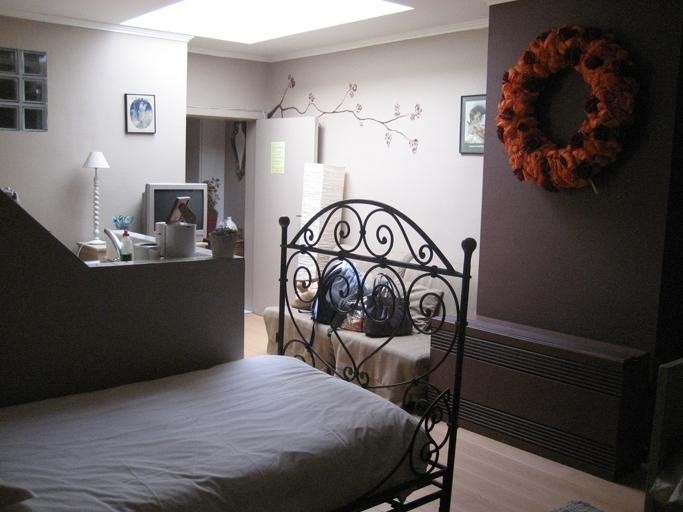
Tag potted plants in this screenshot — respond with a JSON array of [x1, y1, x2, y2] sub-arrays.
[[202, 178, 222, 236], [208, 227, 237, 259]]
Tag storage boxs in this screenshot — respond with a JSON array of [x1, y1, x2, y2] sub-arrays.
[[76, 241, 107, 262]]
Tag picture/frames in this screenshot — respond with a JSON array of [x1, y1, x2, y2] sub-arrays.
[[124, 93, 156, 134], [459, 94, 486, 155]]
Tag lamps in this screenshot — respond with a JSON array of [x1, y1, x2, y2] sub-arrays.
[[81, 150, 111, 245]]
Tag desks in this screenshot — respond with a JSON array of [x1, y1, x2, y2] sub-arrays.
[[204, 238, 244, 258]]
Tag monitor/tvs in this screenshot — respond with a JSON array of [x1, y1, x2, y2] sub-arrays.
[[146, 181, 208, 240]]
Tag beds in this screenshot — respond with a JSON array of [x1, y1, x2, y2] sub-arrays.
[[1, 198, 477, 508]]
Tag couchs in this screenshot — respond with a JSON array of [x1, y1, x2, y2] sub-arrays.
[[264, 271, 478, 410]]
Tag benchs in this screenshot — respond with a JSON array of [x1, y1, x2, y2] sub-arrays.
[[427, 313, 647, 486]]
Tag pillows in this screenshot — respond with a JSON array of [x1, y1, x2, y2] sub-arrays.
[[291, 282, 318, 311], [398, 281, 443, 334]]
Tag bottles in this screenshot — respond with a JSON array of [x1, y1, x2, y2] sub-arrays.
[[120, 229, 134, 262]]
